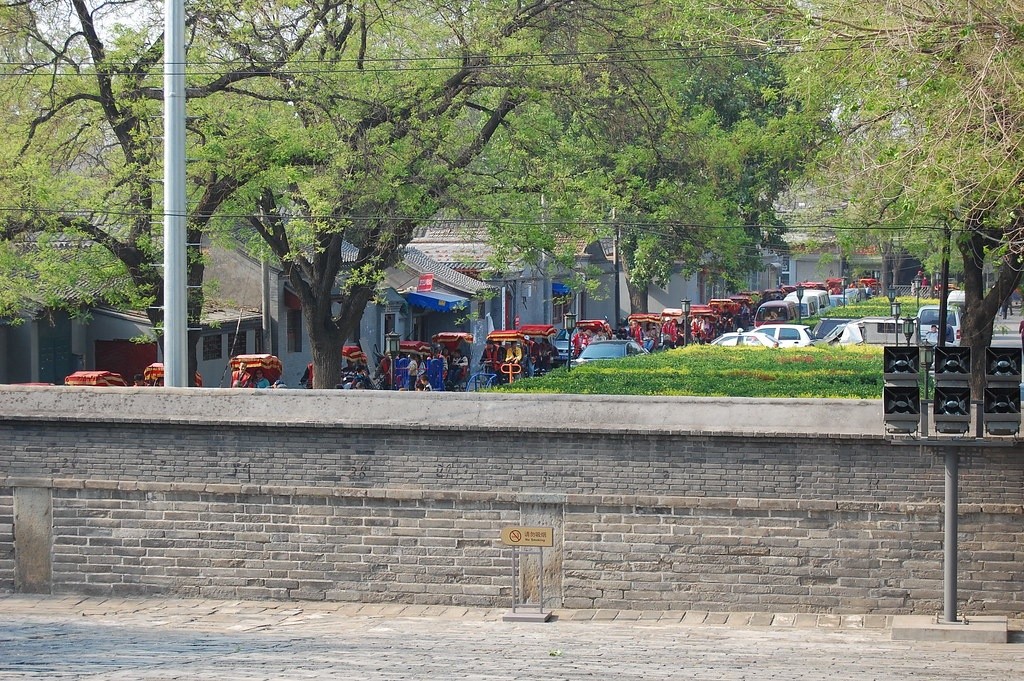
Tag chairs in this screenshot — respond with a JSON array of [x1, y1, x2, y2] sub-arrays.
[[925, 311, 936, 321]]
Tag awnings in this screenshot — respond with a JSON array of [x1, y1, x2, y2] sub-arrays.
[[408, 292, 469, 312], [552, 283, 571, 295]]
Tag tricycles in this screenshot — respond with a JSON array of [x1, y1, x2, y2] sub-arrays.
[[298, 278, 884, 392], [228, 354, 289, 389]]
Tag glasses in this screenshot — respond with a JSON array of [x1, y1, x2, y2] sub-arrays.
[[256, 372, 262, 375]]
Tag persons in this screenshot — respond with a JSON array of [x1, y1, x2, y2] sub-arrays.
[[496, 341, 507, 362], [519, 335, 539, 378], [535, 338, 555, 371], [925, 323, 954, 345], [911, 280, 916, 297], [421, 375, 432, 391], [446, 349, 468, 386], [480, 340, 506, 385], [341, 359, 355, 372], [406, 354, 425, 391], [999, 297, 1013, 319], [253, 369, 270, 388], [373, 352, 391, 390], [572, 280, 872, 359], [231, 362, 252, 388], [442, 347, 453, 365], [299, 363, 313, 389], [133, 374, 149, 386], [505, 341, 522, 364], [350, 368, 370, 389]]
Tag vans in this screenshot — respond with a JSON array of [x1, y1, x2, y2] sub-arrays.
[[947, 290, 966, 314], [913, 304, 963, 348], [751, 299, 798, 331], [783, 289, 831, 321]]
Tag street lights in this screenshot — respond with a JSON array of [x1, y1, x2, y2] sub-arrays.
[[794, 283, 805, 322], [873, 269, 881, 298], [679, 296, 692, 347], [841, 274, 849, 309], [934, 270, 939, 299], [900, 314, 915, 346], [890, 298, 902, 347], [563, 309, 579, 373], [913, 274, 923, 309]]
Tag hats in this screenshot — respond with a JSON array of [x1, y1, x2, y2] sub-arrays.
[[665, 317, 670, 321]]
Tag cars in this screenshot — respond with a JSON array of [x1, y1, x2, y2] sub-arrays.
[[748, 323, 817, 349], [707, 327, 784, 349], [570, 338, 648, 373], [551, 328, 580, 369], [841, 287, 865, 302], [828, 294, 849, 309]]
